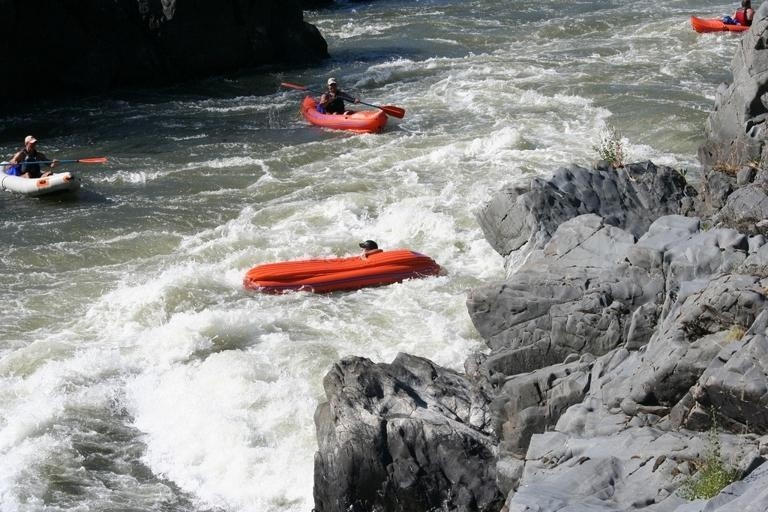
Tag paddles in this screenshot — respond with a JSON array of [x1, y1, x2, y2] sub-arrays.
[[0, 156, 107, 164], [281, 82, 405, 118]]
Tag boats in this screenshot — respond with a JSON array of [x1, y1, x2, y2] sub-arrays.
[[301, 95, 389, 134], [690, 12, 749, 33], [0, 160, 80, 194], [244, 249, 440, 293]]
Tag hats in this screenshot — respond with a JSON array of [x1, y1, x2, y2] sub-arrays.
[[359, 240, 378, 249], [327, 77, 337, 86], [25, 135, 37, 145]]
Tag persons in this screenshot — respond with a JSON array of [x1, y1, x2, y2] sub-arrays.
[[729, 0, 755, 27], [317, 76, 360, 115], [9, 134, 58, 178], [358, 240, 384, 261]]
